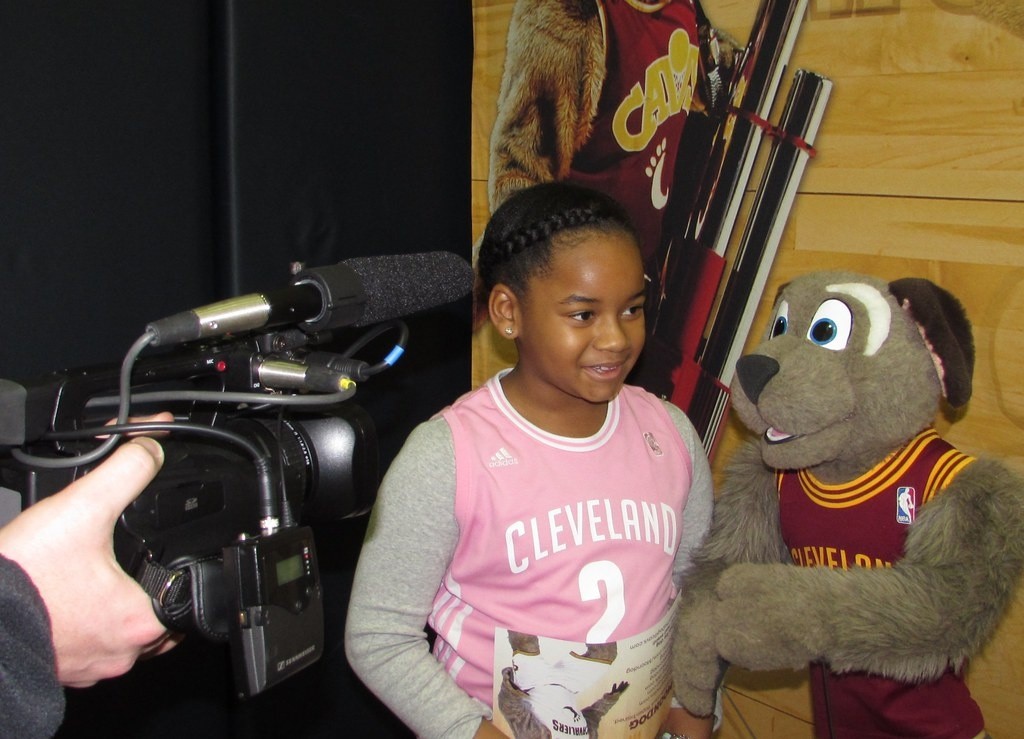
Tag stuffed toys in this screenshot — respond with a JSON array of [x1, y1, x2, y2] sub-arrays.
[[665, 269, 1024, 739]]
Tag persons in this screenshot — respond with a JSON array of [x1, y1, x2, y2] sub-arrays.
[[1, 411, 188, 739], [346, 179, 716, 739]]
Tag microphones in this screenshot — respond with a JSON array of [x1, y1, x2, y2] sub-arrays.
[[145, 250, 476, 347]]
